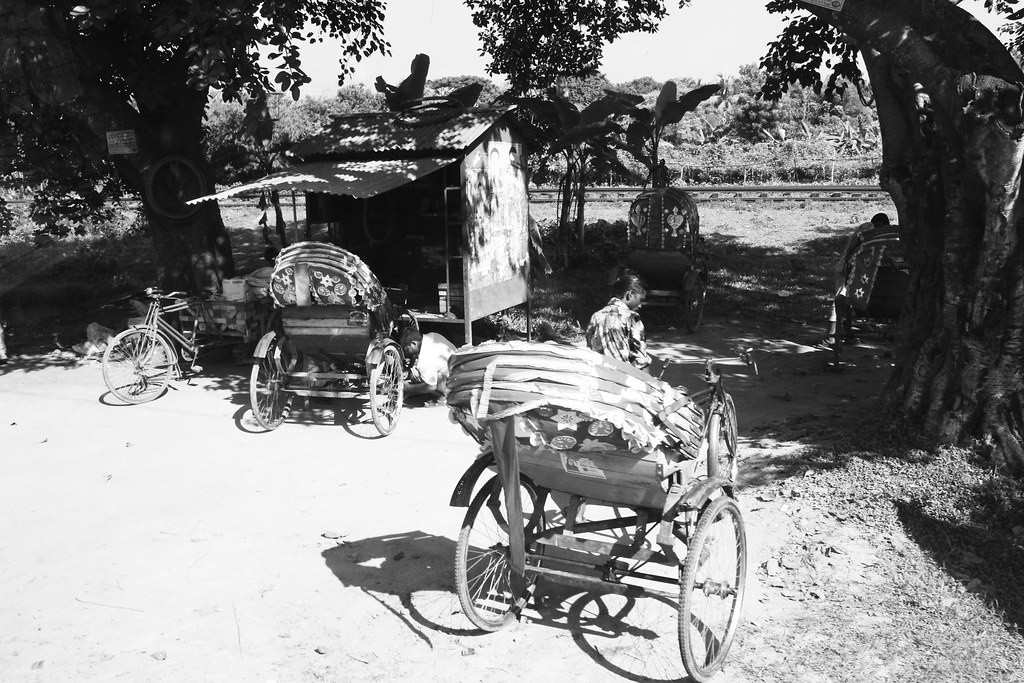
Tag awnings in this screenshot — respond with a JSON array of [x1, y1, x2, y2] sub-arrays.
[[184, 159, 458, 204]]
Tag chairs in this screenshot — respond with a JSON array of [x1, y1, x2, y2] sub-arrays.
[[624, 186, 710, 285], [843, 223, 910, 315], [268, 237, 404, 358], [446, 325, 712, 513]]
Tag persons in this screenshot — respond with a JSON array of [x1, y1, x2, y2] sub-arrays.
[[828, 213, 890, 346], [656, 158, 671, 188], [586, 274, 652, 374]]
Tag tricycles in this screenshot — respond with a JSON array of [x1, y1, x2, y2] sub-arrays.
[[615, 188, 712, 332], [828, 219, 909, 360], [242, 239, 422, 438], [416, 331, 761, 683], [101, 282, 285, 405]]
